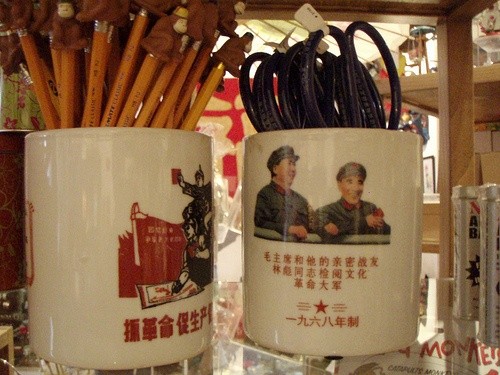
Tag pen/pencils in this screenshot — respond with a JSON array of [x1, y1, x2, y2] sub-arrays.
[[2, 1, 254, 130]]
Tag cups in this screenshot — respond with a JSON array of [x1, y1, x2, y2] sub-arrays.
[[23, 127, 215, 370], [242, 127, 423, 358]]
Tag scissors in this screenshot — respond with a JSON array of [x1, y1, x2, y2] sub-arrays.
[[238, 19, 402, 134]]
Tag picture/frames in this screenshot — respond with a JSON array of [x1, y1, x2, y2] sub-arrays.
[[423, 155, 436, 193]]
